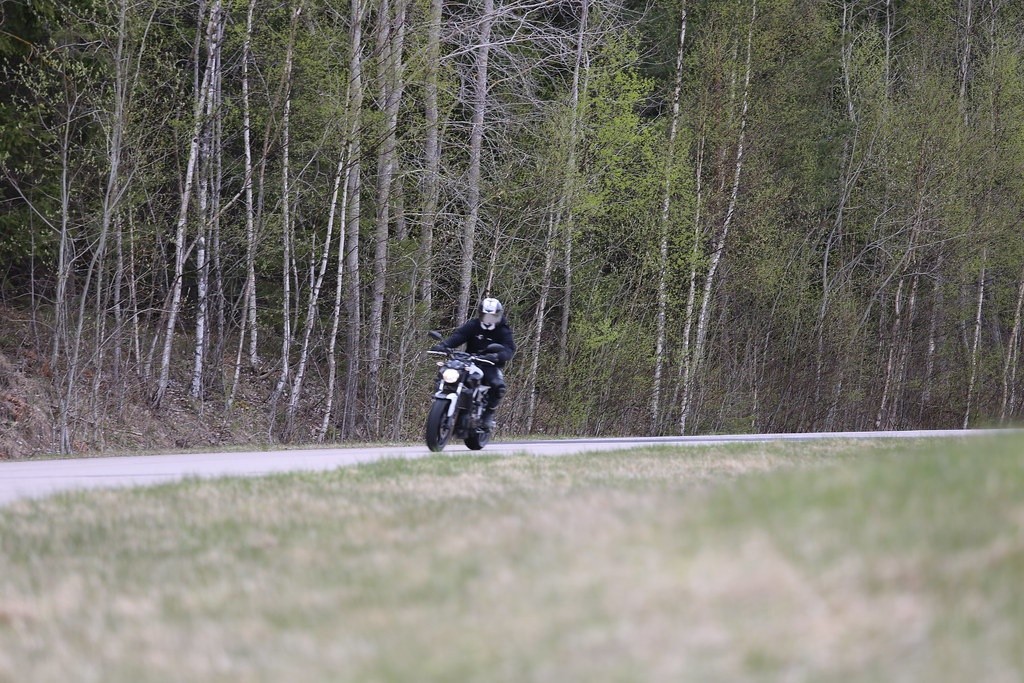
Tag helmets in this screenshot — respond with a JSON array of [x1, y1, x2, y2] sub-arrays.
[[478, 298, 504, 331]]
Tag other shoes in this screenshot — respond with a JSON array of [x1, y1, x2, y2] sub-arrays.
[[481, 411, 496, 429]]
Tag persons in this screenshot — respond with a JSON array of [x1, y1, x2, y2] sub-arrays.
[[430, 297, 516, 428]]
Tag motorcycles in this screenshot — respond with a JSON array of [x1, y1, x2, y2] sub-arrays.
[[426, 330, 506, 452]]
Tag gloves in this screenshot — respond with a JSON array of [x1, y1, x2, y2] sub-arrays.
[[430, 344, 449, 357], [479, 353, 499, 367]]
